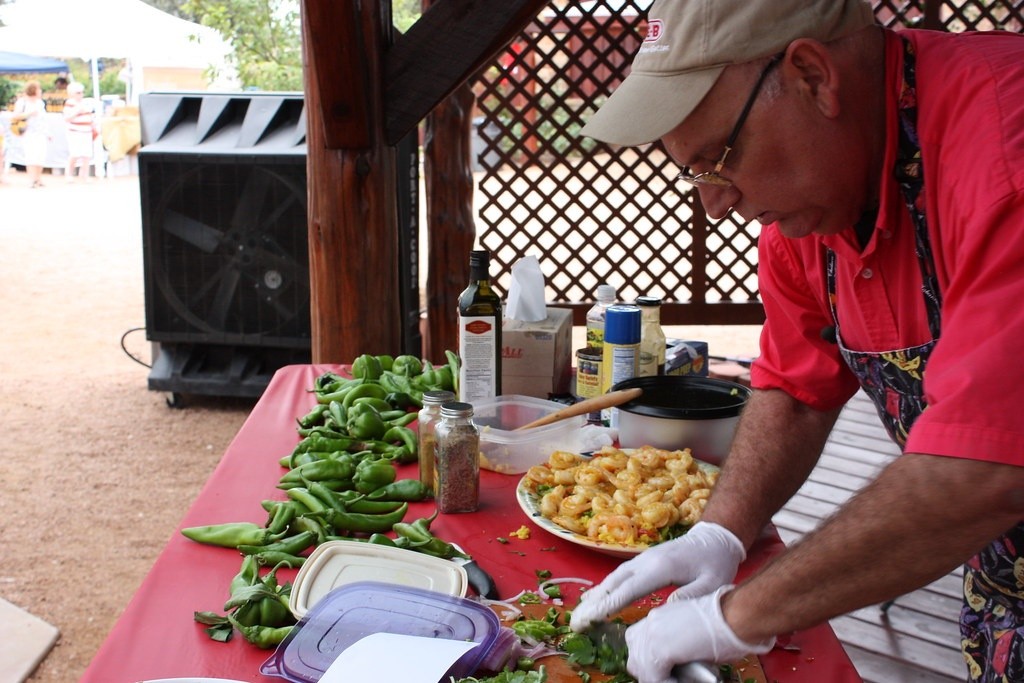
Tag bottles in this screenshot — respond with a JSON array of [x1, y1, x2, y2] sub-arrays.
[[636, 297, 666, 376], [434, 402, 479, 513], [457, 249, 502, 428], [585, 285, 616, 347], [417, 391, 456, 491]]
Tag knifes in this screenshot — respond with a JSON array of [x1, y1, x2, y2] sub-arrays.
[[587, 623, 721, 683]]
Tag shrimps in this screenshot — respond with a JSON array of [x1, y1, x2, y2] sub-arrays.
[[528, 445, 719, 545]]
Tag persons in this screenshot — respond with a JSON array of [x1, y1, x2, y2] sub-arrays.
[[61, 81, 93, 179], [570, 0, 1024, 683], [13, 81, 50, 189], [45, 76, 72, 113]]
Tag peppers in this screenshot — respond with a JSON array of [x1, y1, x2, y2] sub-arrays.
[[179, 351, 472, 648]]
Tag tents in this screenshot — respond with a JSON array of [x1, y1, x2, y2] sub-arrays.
[[0, 1, 222, 77]]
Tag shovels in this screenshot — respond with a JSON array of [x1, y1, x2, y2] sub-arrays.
[[478, 387, 641, 452]]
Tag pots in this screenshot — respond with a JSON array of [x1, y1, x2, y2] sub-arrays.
[[606, 375, 753, 465]]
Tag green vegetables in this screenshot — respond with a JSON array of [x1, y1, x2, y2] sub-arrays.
[[449, 518, 760, 683]]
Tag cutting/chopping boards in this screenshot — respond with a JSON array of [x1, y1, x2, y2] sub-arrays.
[[478, 600, 767, 683]]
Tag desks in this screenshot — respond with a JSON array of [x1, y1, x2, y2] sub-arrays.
[[74, 365, 861, 683]]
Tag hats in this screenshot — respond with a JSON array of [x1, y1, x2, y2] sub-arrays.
[[579, 0, 875, 147]]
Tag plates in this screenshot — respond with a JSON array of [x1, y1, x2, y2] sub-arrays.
[[515, 448, 721, 558]]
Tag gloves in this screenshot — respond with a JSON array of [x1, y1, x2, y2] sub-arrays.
[[625, 584, 777, 683], [570, 521, 747, 634]]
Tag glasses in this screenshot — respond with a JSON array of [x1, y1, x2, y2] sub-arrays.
[[678, 43, 792, 193]]
